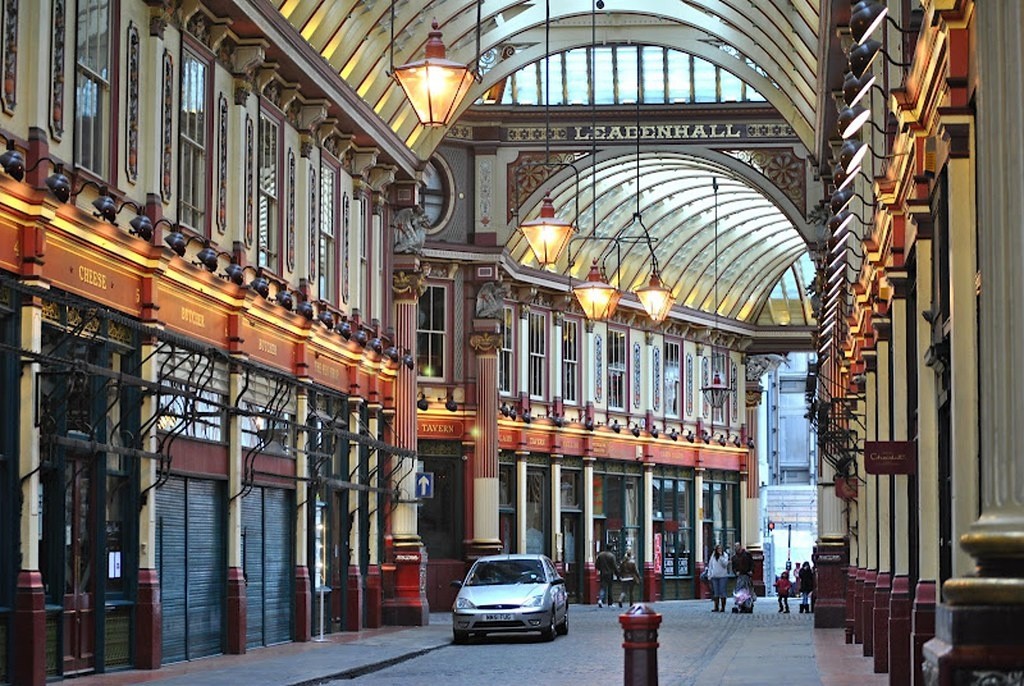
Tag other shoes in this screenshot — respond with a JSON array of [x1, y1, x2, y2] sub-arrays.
[[618, 599, 623, 608], [608, 605, 616, 609], [779, 609, 783, 612], [784, 611, 789, 613], [598, 600, 603, 608]]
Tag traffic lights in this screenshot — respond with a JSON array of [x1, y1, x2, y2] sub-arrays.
[[768, 522, 775, 531]]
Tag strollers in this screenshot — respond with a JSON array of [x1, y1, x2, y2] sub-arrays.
[[731, 572, 757, 614]]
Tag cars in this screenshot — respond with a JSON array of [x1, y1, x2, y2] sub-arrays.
[[450, 553, 568, 644]]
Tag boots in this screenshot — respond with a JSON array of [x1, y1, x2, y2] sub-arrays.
[[711, 597, 719, 612], [800, 604, 804, 614], [720, 597, 726, 612], [804, 604, 810, 613]]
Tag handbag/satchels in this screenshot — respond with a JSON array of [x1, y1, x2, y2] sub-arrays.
[[699, 567, 709, 583]]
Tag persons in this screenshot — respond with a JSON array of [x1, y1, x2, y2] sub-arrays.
[[773, 570, 791, 613], [708, 545, 728, 612], [798, 561, 813, 613], [595, 543, 640, 608], [731, 542, 756, 602]]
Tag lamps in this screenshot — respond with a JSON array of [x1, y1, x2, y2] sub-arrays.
[[804, 0, 920, 494], [415, 391, 756, 450], [1, 132, 414, 372], [695, 177, 732, 408], [512, 0, 581, 265], [567, 0, 624, 321], [633, 43, 676, 322], [384, 0, 484, 130]]
